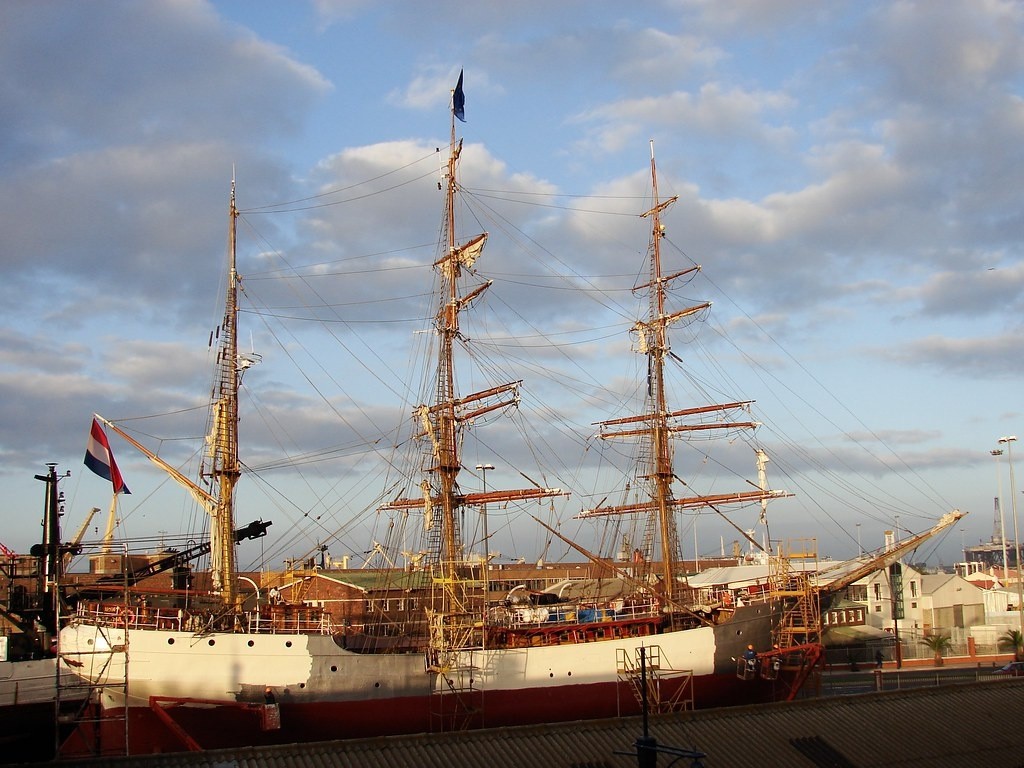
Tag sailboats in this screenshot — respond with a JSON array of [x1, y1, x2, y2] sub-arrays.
[[44, 64, 972, 755]]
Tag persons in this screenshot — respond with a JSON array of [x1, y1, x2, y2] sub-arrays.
[[769, 644, 784, 678], [875, 649, 885, 669], [744, 644, 757, 671]]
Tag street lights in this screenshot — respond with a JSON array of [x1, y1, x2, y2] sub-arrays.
[[894, 515, 901, 543], [989, 448, 1009, 590], [856, 522, 862, 558], [997, 434, 1024, 643], [475, 463, 497, 623], [959, 528, 968, 561]]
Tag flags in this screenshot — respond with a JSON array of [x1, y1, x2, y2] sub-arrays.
[[448, 67, 466, 123], [83, 414, 132, 494]]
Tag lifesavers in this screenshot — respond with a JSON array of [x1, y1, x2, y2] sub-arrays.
[[118, 610, 135, 624]]
